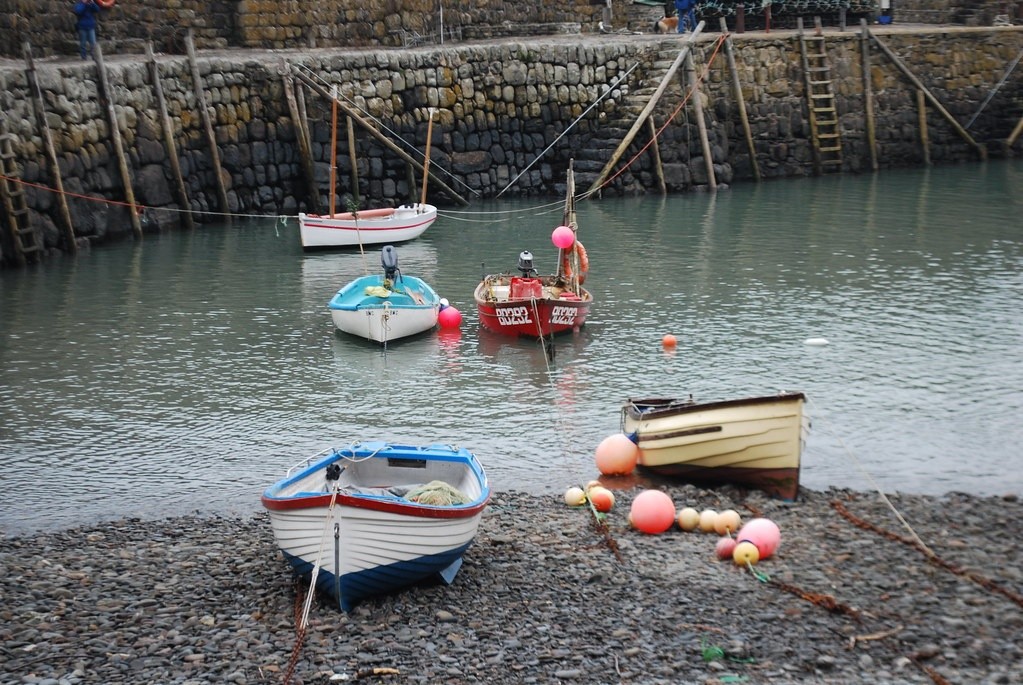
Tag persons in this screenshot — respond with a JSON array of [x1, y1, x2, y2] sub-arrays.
[[73, 0, 99, 62], [675, 0, 698, 34]]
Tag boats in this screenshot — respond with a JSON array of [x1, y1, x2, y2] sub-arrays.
[[621, 393, 809, 502], [474, 250, 594, 338], [261, 441, 491, 612], [329, 246, 441, 342], [298, 203, 438, 254]]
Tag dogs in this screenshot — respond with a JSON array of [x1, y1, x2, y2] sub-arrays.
[[657, 16, 679, 35]]
